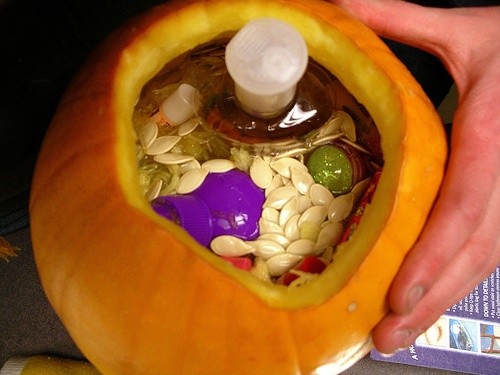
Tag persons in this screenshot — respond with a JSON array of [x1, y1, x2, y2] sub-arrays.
[[319, 1, 499, 354]]
